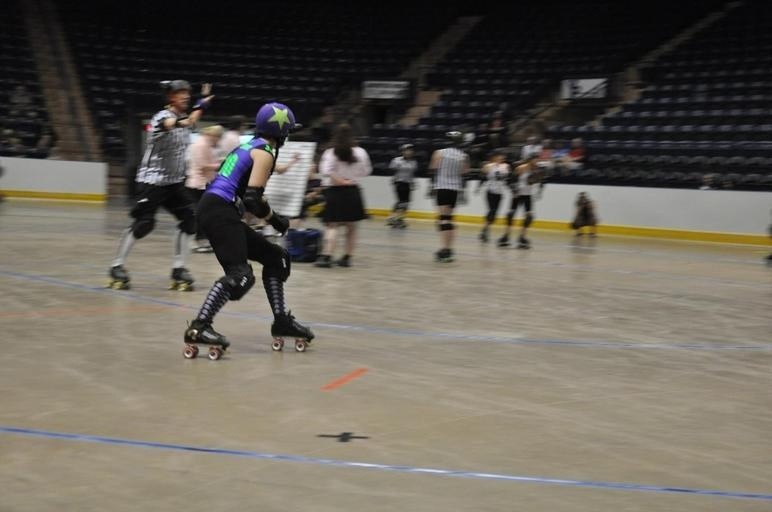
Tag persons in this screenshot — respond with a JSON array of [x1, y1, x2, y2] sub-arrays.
[[316, 121, 372, 268], [572, 192, 596, 237], [185, 115, 300, 226], [184, 102, 314, 360], [475, 147, 539, 249], [3, 85, 50, 150], [427, 132, 470, 265], [387, 143, 418, 230], [522, 136, 584, 183], [107, 81, 214, 288]]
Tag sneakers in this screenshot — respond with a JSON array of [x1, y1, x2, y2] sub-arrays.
[[589, 232, 598, 239], [336, 253, 354, 267], [576, 232, 585, 238], [315, 253, 333, 269]]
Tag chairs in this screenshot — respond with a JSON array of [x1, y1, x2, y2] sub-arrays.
[[0, 1, 772, 193]]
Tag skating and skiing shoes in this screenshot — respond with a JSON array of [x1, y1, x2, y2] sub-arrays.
[[496, 232, 512, 249], [434, 248, 454, 265], [386, 214, 409, 231], [270, 314, 316, 353], [107, 264, 132, 292], [168, 267, 197, 294], [182, 321, 230, 360], [515, 235, 533, 250]]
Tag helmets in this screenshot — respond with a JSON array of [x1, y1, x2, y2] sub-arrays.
[[164, 78, 193, 97], [400, 142, 415, 151], [446, 130, 463, 139], [254, 101, 302, 147]]
